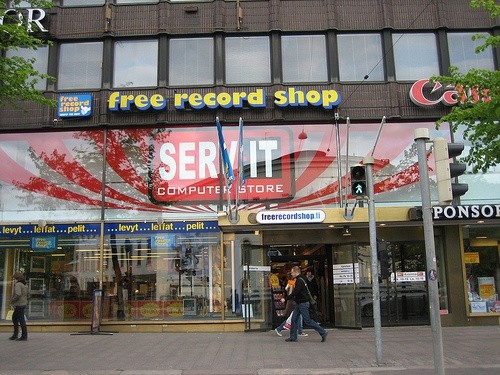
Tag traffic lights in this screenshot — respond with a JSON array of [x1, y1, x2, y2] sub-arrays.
[[434, 137, 471, 203], [350, 166, 368, 198]]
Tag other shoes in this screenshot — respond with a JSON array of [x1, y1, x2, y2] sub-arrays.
[[275, 329, 283, 337], [9, 335, 18, 340], [16, 336, 27, 341], [298, 333, 309, 337], [285, 338, 297, 342], [321, 331, 328, 342]]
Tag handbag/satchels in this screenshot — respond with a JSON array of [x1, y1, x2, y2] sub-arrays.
[[310, 297, 318, 305], [6, 309, 15, 321], [283, 311, 294, 331]]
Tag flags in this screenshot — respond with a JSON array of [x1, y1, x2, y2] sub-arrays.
[[216, 121, 245, 186]]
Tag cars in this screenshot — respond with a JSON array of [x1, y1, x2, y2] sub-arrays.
[[361, 289, 428, 317]]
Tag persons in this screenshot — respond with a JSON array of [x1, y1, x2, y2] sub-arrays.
[[66, 277, 84, 301], [9, 271, 29, 341], [306, 268, 322, 325], [274, 272, 309, 337], [280, 266, 328, 342]]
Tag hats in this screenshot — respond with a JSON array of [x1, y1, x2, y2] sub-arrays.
[[13, 272, 25, 282]]
